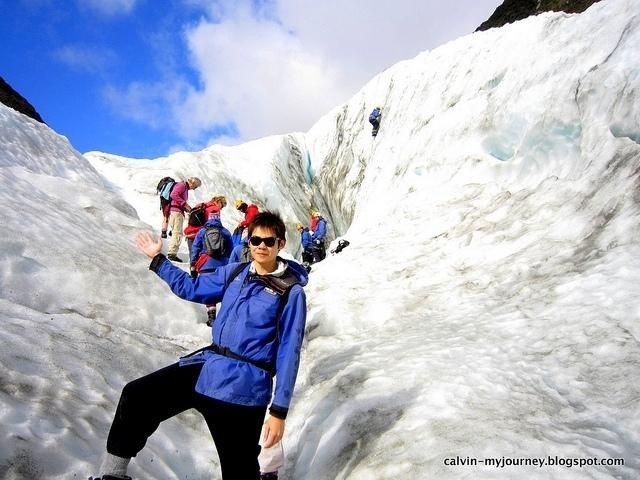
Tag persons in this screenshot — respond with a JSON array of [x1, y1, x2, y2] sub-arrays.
[[183, 195, 227, 262], [168, 176, 202, 262], [296, 224, 314, 265], [369, 106, 381, 136], [190, 209, 234, 327], [94, 206, 309, 479], [235, 200, 259, 229], [161, 203, 172, 238], [312, 212, 328, 263]]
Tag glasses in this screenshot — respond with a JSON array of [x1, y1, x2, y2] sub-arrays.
[[248, 237, 281, 247]]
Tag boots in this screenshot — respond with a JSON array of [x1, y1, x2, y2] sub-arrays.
[[206, 310, 216, 326]]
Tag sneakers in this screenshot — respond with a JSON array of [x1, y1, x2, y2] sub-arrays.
[[162, 231, 172, 238]]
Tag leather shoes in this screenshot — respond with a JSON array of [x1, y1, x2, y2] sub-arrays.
[[168, 254, 183, 262]]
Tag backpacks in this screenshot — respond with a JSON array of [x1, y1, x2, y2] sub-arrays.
[[188, 203, 206, 226], [157, 177, 186, 210], [203, 227, 228, 259]]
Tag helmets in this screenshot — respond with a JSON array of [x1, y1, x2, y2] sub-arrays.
[[236, 200, 245, 209], [298, 224, 303, 230], [314, 212, 321, 217]]
